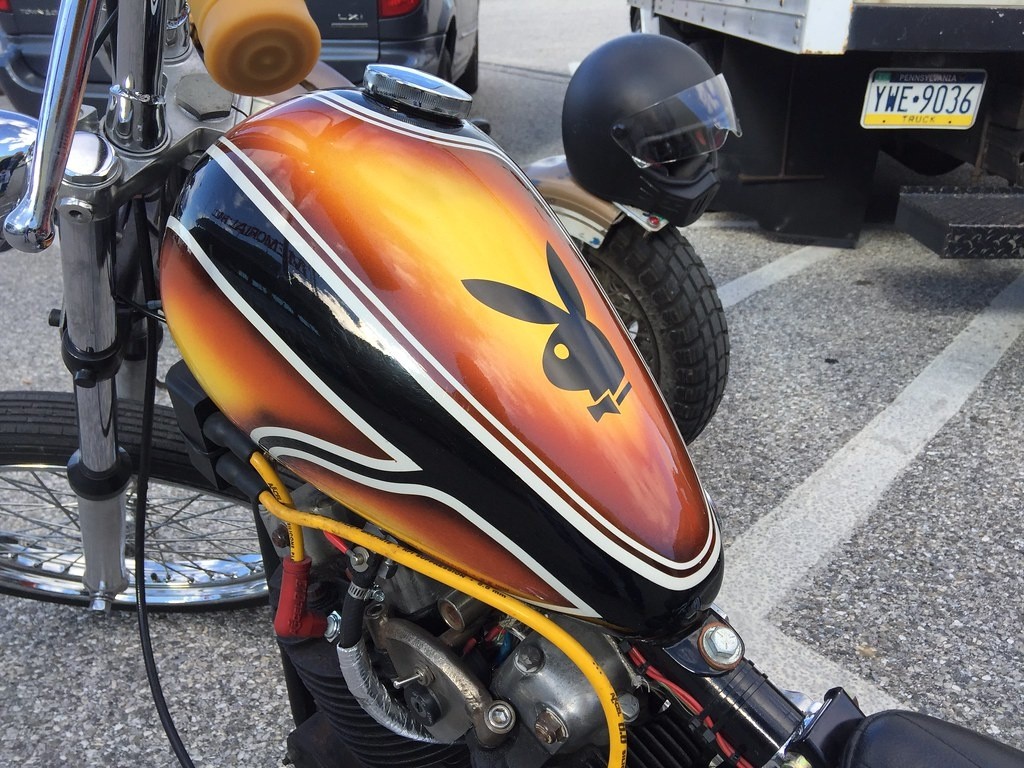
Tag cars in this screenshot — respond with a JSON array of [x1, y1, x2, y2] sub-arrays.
[[0, 3, 481, 124]]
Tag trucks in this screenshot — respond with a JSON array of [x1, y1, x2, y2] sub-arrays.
[[629, 1, 1024, 261]]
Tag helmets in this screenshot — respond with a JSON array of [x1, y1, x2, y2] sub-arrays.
[[561, 33, 744, 228]]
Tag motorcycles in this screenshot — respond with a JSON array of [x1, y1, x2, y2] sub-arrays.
[[0, 1, 1024, 768]]
